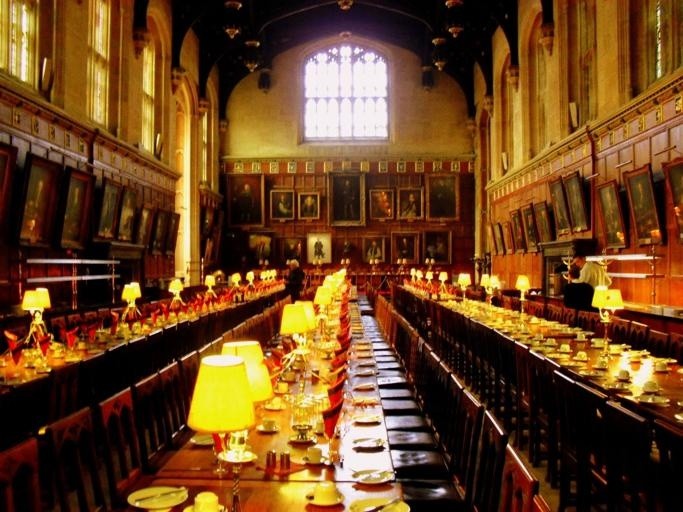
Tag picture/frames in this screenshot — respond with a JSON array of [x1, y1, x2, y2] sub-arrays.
[[0, 142, 181, 258], [218, 160, 472, 265], [485, 157, 683, 257]]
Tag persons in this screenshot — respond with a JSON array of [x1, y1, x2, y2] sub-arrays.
[[65, 185, 82, 218], [287, 239, 295, 257], [634, 180, 650, 217], [301, 196, 316, 217], [603, 186, 619, 234], [97, 191, 114, 237], [119, 198, 133, 240], [239, 183, 251, 201], [313, 237, 322, 259], [570, 182, 584, 226], [340, 179, 354, 219], [538, 209, 548, 240], [514, 216, 523, 249], [400, 192, 416, 216], [567, 252, 611, 290], [367, 240, 381, 258], [430, 178, 455, 218], [31, 179, 43, 201], [273, 192, 291, 217], [279, 259, 305, 303], [559, 265, 598, 313], [295, 240, 303, 258], [397, 236, 413, 259]]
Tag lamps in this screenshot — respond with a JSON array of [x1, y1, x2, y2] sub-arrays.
[[419, 0, 462, 89], [219, 0, 276, 96]]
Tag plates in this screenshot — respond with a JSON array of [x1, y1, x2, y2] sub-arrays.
[[436, 300, 683, 429], [0, 280, 409, 511]]
